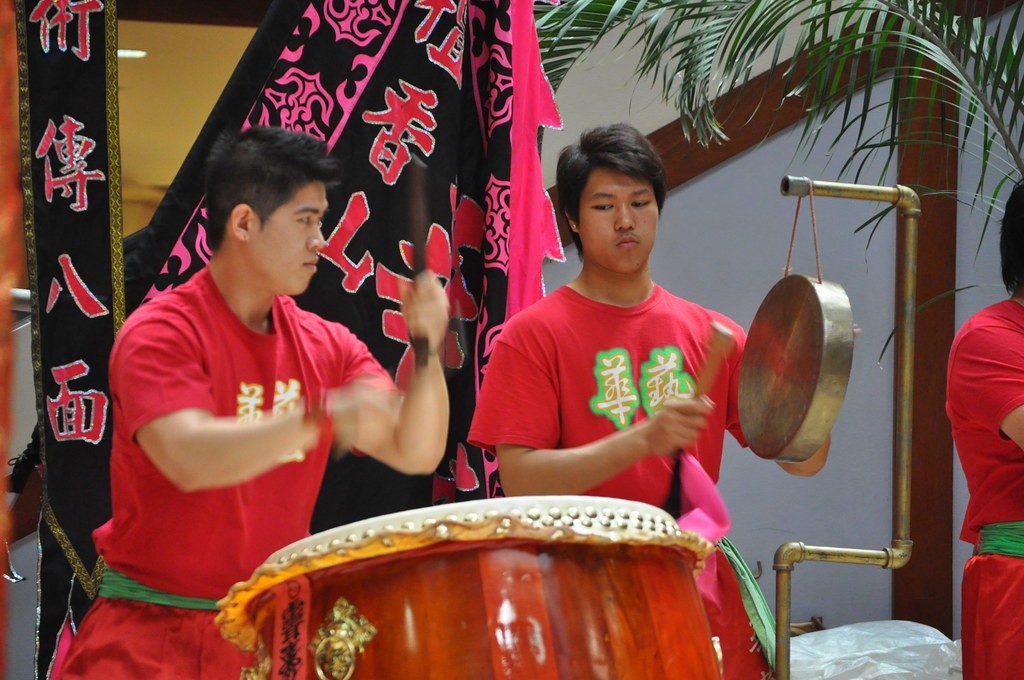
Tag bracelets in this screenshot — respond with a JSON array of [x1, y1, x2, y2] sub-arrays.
[[430, 349, 438, 357]]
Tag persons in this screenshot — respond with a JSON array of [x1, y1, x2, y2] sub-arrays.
[[946, 177, 1024, 680], [60, 123, 451, 680], [466, 121, 862, 680]]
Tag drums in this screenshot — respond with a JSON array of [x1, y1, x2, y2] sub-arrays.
[[217, 496, 728, 680]]
